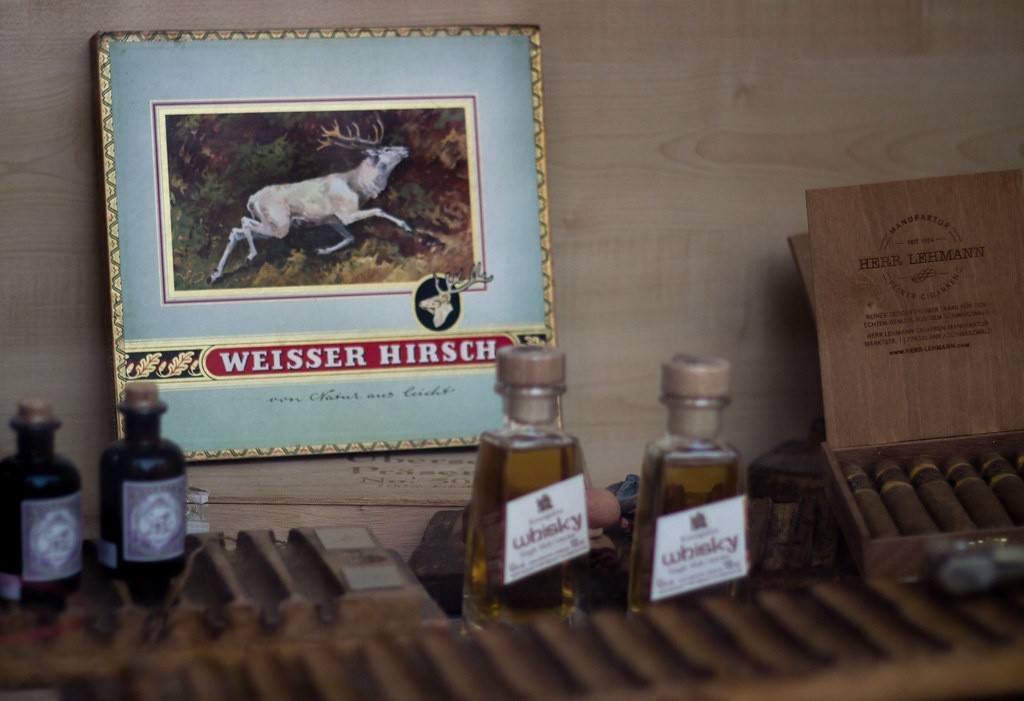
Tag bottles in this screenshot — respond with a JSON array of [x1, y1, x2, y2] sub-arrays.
[[96, 384, 187, 582], [464, 346, 587, 629], [628, 354, 749, 614], [1, 401, 82, 602]]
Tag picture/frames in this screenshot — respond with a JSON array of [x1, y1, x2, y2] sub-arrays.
[[89, 23, 562, 462]]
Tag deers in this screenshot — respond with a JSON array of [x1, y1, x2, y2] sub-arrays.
[[207, 116, 413, 284]]
